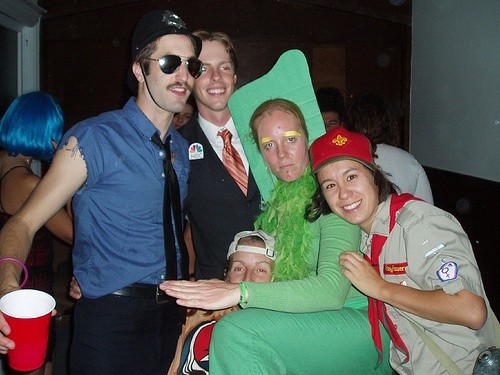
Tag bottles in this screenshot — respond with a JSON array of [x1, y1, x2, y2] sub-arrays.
[[473, 349, 500, 375]]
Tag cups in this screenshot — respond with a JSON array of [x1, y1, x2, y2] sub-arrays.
[[0, 289, 56, 371]]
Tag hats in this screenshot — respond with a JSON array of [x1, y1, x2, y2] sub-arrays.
[[226, 229, 277, 262], [126, 8, 202, 85], [307, 126, 377, 176]]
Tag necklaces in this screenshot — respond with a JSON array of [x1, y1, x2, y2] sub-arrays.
[[26, 159, 30, 167]]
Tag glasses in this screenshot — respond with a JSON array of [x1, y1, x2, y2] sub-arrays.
[[140, 53, 205, 80]]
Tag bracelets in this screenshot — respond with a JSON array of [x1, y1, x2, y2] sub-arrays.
[[238, 282, 246, 305], [0, 257, 30, 287]]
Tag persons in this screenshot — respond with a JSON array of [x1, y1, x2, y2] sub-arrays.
[[160, 49, 394, 375], [179, 30, 268, 282], [0, 9, 203, 375], [304, 126, 500, 375], [70, 88, 435, 375], [0, 91, 74, 375]]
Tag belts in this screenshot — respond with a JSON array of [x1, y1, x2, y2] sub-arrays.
[[109, 282, 178, 305]]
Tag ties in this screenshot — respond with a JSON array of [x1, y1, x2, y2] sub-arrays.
[[216, 127, 249, 198], [151, 131, 191, 282], [363, 191, 427, 366]]
